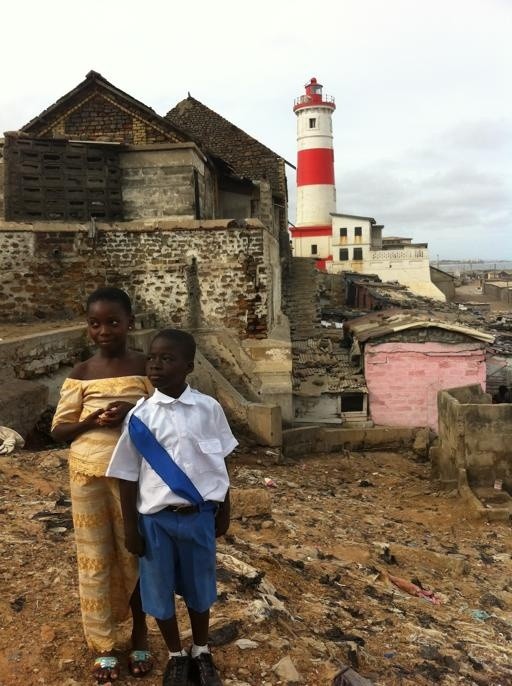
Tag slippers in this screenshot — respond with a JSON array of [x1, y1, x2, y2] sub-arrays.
[[94, 651, 152, 681]]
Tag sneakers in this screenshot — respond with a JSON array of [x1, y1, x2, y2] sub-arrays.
[[164, 653, 223, 686]]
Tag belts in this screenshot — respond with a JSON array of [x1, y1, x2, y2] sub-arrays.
[[169, 505, 199, 514]]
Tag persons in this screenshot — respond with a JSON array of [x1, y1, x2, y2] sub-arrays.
[[50, 285, 157, 683], [104, 327, 242, 686]]
